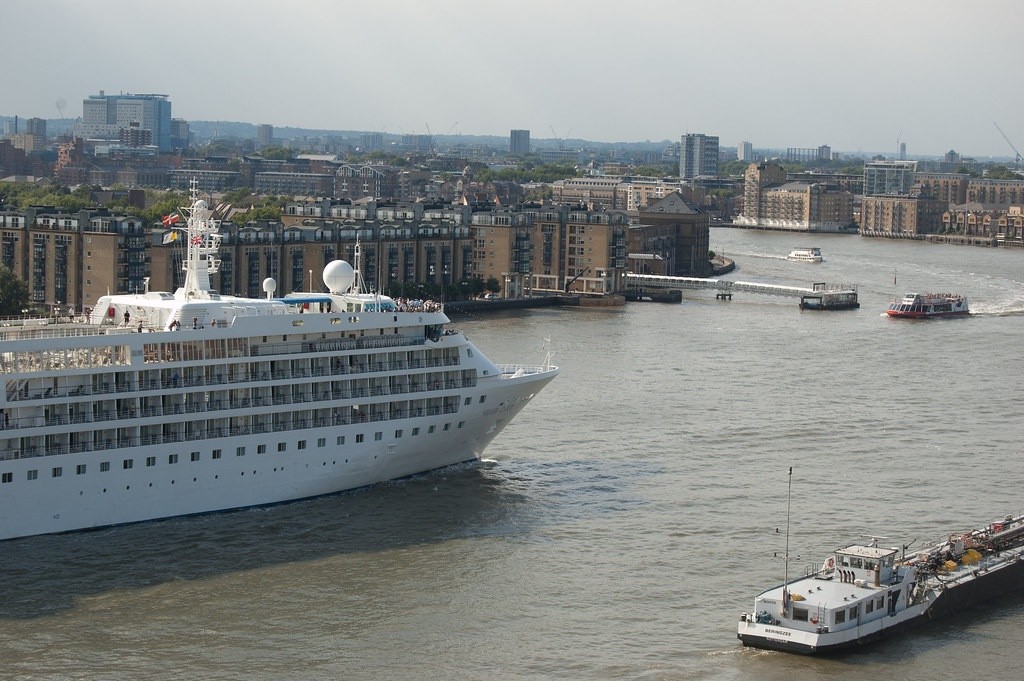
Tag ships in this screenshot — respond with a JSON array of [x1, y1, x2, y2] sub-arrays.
[[2, 185, 561, 546]]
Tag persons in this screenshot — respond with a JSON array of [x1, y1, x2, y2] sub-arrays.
[[175, 321, 182, 331], [387, 294, 458, 342], [210, 318, 216, 328], [172, 372, 181, 389], [915, 292, 966, 303], [192, 316, 198, 329], [168, 320, 175, 332], [356, 410, 366, 424], [336, 414, 346, 424], [83, 308, 93, 325], [137, 319, 143, 332], [67, 306, 77, 323], [4, 412, 10, 430], [336, 358, 344, 373], [434, 378, 440, 391], [123, 310, 131, 326]]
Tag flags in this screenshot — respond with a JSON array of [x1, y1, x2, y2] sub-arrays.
[[162, 231, 177, 245], [190, 235, 205, 247], [162, 210, 180, 228]]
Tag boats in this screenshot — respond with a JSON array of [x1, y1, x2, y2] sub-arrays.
[[738, 465, 1024, 659], [798, 287, 861, 311], [788, 246, 824, 263], [886, 291, 972, 319]]
